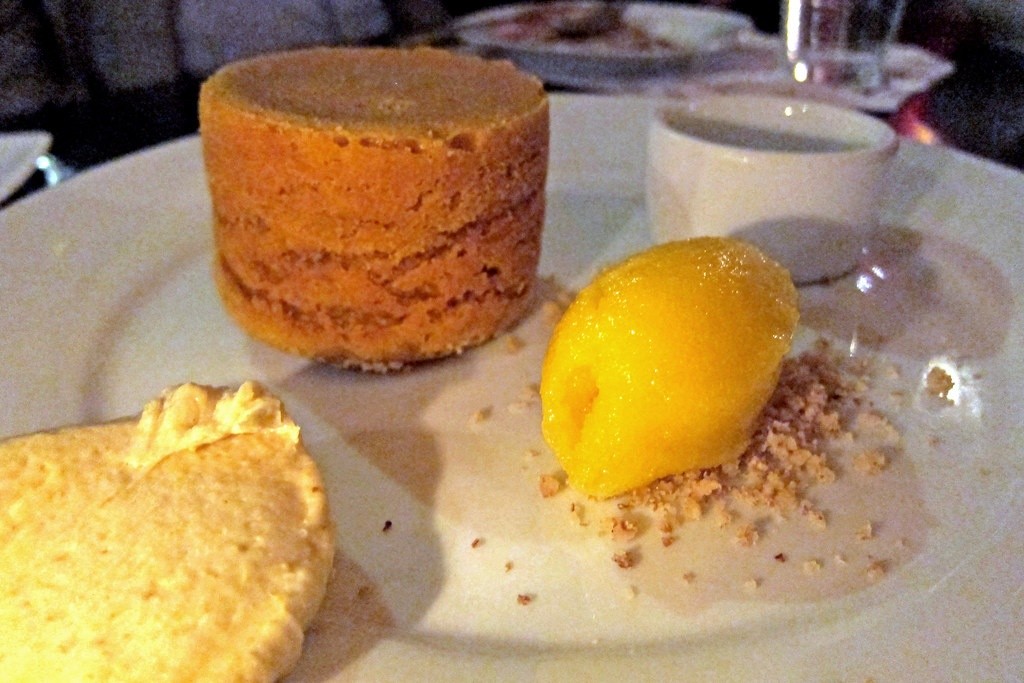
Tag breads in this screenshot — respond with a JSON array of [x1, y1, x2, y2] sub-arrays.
[[200, 46, 548, 367]]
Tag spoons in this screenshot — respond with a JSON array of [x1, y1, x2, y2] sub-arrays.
[[398, 3, 626, 46]]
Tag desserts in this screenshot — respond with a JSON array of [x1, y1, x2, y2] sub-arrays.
[[540, 234, 802, 501]]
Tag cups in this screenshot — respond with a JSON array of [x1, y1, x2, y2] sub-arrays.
[[780, 0, 905, 96]]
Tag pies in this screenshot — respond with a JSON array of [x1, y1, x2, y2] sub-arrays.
[[0, 374, 338, 683]]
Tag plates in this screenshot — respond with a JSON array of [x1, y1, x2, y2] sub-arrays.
[[0, 92, 1024, 682], [0, 131, 53, 203], [451, 1, 751, 72]]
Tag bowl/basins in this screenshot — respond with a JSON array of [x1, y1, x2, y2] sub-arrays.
[[645, 93, 899, 283]]
